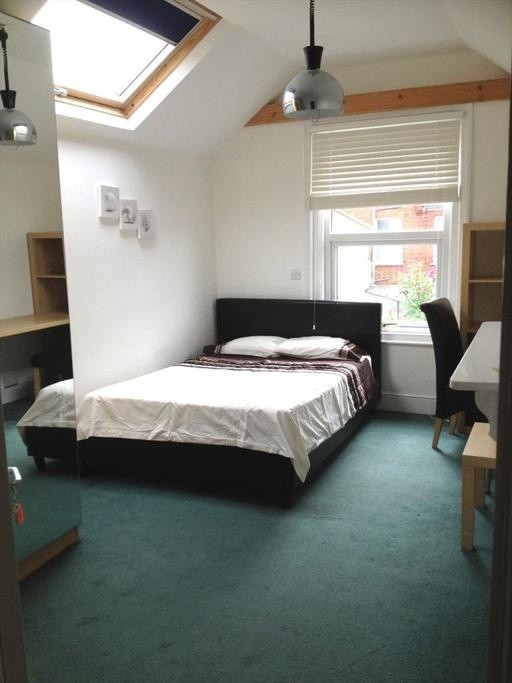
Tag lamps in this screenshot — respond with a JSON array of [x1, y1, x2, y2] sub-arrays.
[[281, 0, 346, 123], [0, 22, 39, 148]]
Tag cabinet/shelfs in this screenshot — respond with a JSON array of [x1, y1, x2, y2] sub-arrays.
[[460, 220, 506, 361], [25, 231, 69, 384]]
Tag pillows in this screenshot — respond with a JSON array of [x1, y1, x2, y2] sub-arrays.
[[203, 331, 368, 363]]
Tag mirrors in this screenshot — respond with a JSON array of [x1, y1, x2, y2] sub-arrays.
[[0, 9, 84, 565]]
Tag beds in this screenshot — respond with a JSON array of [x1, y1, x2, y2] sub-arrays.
[[73, 296, 382, 510]]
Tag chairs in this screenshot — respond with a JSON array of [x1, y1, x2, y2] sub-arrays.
[[419, 296, 476, 450]]
